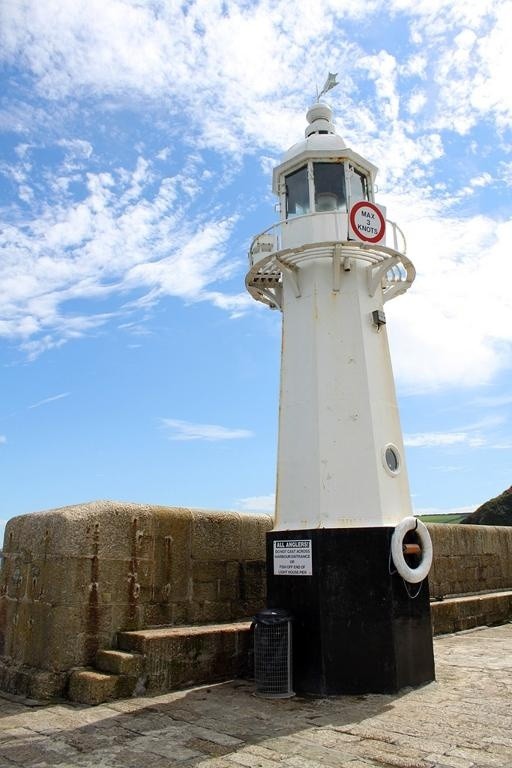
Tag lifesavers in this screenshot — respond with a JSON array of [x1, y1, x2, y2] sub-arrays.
[[391, 517, 432, 582]]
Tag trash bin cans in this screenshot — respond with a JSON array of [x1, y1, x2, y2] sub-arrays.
[[250, 608, 296, 699]]
